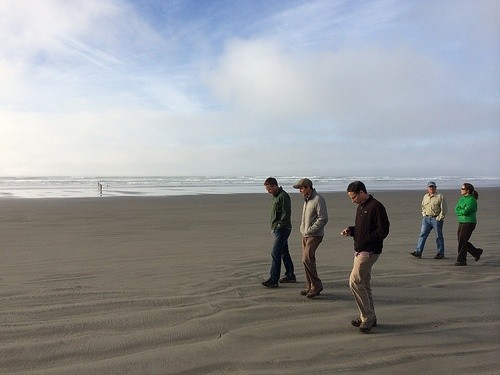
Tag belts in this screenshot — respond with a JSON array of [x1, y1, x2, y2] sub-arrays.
[[426, 215, 437, 217]]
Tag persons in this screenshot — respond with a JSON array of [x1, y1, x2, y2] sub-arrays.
[[342, 181, 390, 332], [454, 183, 483, 267], [262, 177, 296, 287], [293, 178, 323, 297], [412, 182, 447, 259]]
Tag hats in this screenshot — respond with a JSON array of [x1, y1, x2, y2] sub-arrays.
[[428, 182, 436, 187], [293, 178, 312, 189]]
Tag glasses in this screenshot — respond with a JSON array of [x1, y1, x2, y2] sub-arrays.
[[461, 188, 464, 190]]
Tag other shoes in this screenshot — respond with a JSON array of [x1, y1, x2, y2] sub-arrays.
[[360, 316, 375, 332], [411, 251, 421, 257], [279, 277, 296, 283], [300, 289, 320, 295], [434, 253, 444, 258], [262, 281, 278, 286], [475, 249, 483, 261], [306, 288, 323, 297], [455, 262, 466, 266], [351, 315, 376, 327]]
